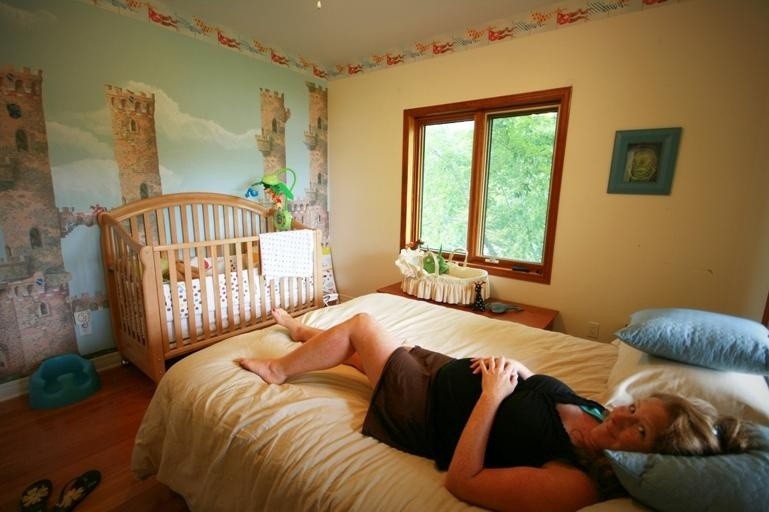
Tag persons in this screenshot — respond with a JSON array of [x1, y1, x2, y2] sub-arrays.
[[236, 307, 749, 511]]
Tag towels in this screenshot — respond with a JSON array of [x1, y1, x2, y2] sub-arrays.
[[257, 227, 315, 287]]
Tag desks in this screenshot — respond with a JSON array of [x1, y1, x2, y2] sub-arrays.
[[377, 280, 560, 331]]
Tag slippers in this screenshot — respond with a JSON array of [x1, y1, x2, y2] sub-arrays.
[[20, 479, 53, 512], [51, 469, 102, 512]]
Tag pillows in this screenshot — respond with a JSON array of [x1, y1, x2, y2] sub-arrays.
[[599, 303, 769, 512], [108, 253, 170, 281]]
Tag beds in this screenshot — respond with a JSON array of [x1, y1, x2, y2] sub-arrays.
[[128, 288, 769, 512], [95, 191, 324, 390]]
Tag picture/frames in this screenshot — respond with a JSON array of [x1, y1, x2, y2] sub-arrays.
[[606, 123, 684, 198]]
